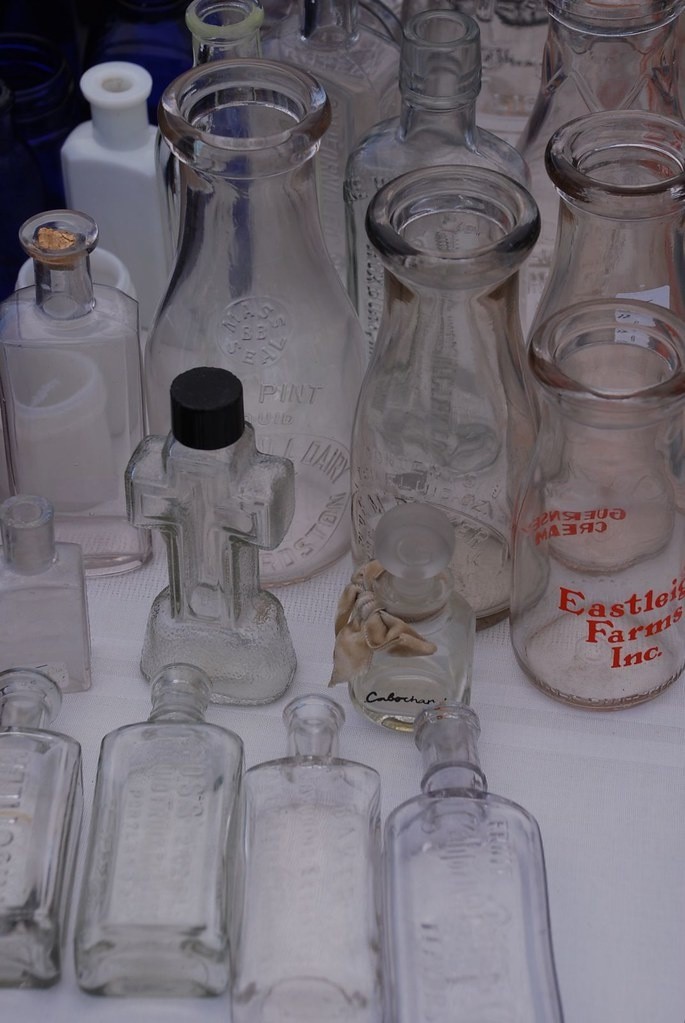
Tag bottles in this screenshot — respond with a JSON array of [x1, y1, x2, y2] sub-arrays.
[[0, 668, 83, 989], [72, 662, 243, 998], [232, 695, 384, 1023], [0, 0, 685, 735], [377, 703, 564, 1022]]
